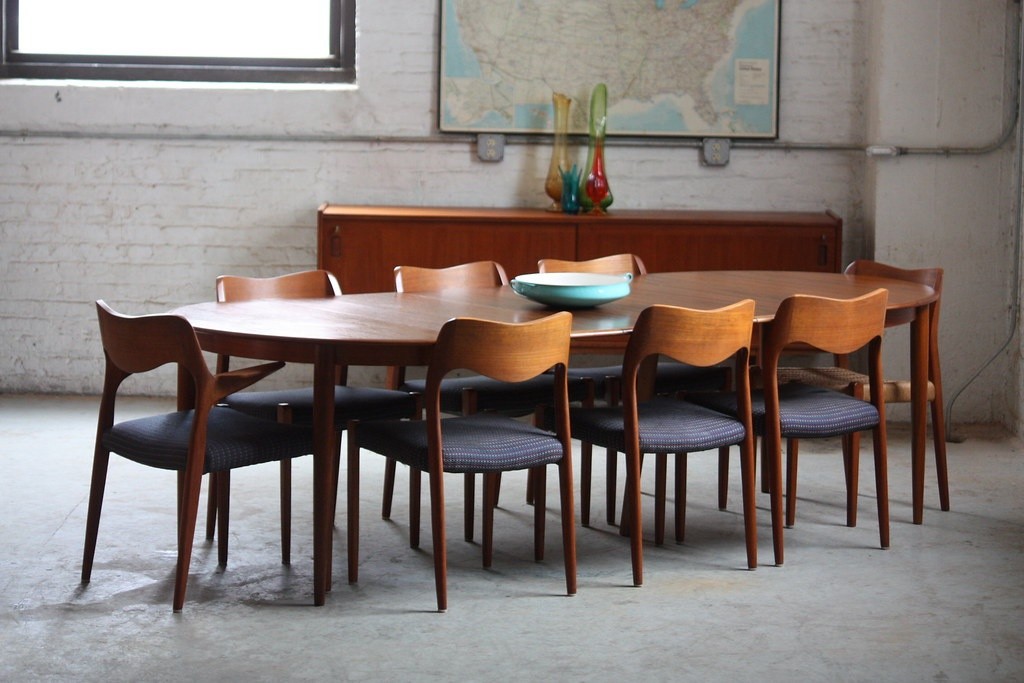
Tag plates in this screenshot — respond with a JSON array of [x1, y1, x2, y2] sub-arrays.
[[510, 271, 633, 306]]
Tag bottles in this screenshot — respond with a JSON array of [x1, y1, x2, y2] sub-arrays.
[[545, 82, 614, 217]]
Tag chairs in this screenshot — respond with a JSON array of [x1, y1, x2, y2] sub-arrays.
[[837, 257, 951, 523], [80, 250, 886, 615]]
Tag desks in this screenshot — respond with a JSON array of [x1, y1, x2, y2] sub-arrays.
[[165, 269, 938, 608], [315, 202, 844, 275]]
